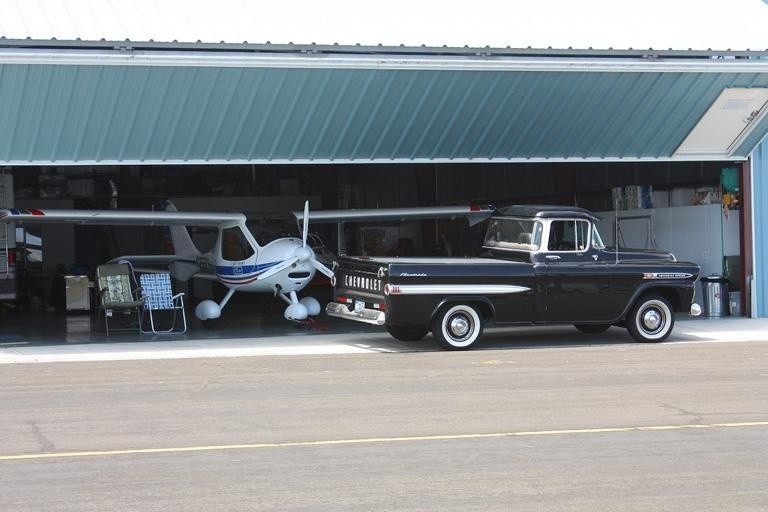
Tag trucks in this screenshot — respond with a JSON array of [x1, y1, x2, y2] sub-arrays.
[[0, 166, 17, 300]]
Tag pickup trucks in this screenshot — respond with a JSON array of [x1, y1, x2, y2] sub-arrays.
[[325, 204, 702, 350]]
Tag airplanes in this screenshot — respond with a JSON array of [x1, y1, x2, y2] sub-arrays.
[[0, 176, 497, 330]]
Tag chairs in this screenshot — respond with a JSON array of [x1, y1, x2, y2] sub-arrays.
[[92, 263, 189, 337]]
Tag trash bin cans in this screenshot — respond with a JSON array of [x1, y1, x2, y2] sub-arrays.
[[700, 273, 728, 317]]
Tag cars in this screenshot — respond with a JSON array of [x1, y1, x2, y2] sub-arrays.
[[17, 243, 41, 300]]
